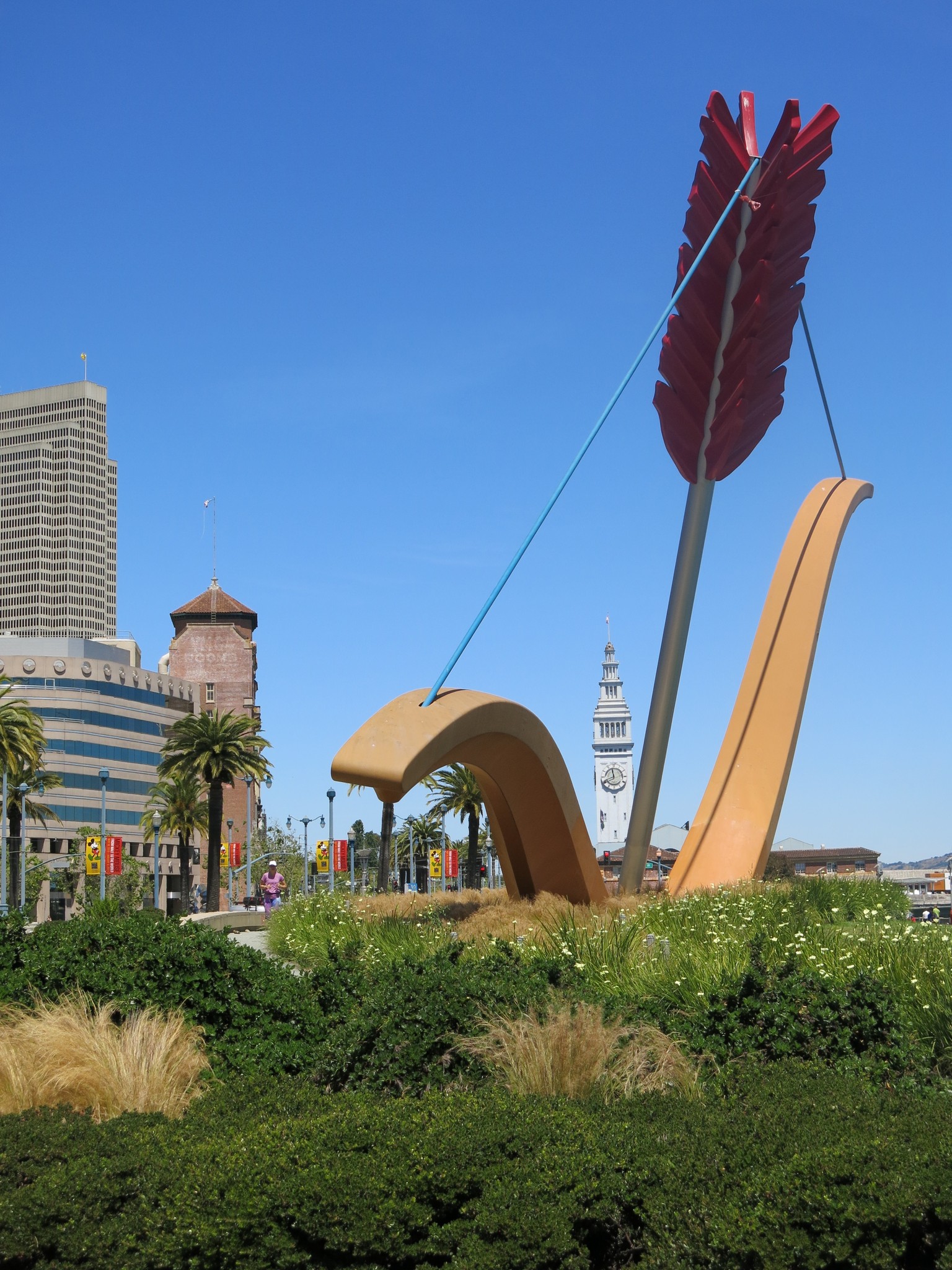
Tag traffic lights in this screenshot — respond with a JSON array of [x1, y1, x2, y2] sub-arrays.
[[264, 850, 272, 863], [603, 851, 611, 866], [447, 885, 451, 889], [479, 865, 485, 877], [279, 850, 286, 863]]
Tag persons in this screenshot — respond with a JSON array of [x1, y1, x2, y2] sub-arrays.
[[928, 907, 938, 923], [907, 913, 916, 922], [259, 860, 288, 921], [922, 908, 930, 923], [932, 905, 940, 924]]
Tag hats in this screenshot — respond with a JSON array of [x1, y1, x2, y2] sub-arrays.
[[269, 861, 277, 866]]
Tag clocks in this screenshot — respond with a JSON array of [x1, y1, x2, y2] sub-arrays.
[[601, 763, 627, 789]]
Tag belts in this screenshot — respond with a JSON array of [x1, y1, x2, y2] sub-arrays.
[[265, 892, 278, 894]]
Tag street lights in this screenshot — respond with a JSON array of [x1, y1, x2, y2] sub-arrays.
[[479, 815, 497, 888], [414, 836, 443, 898], [393, 830, 399, 879], [457, 859, 463, 892], [3, 780, 45, 914], [392, 812, 431, 883], [224, 775, 273, 897], [347, 828, 356, 892], [286, 814, 326, 898], [326, 787, 335, 892], [439, 802, 448, 892], [226, 817, 233, 910], [98, 765, 110, 902], [656, 848, 662, 887], [152, 807, 162, 908]]
